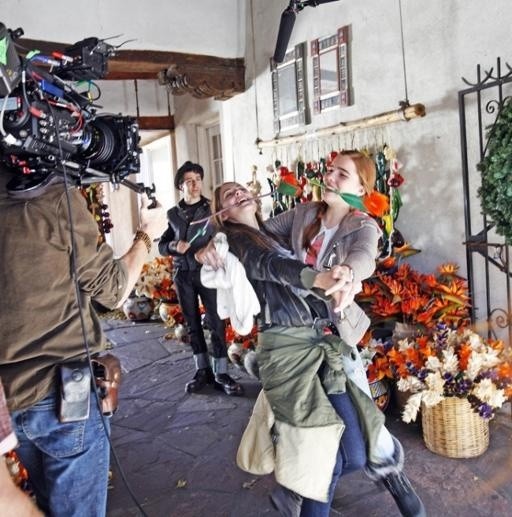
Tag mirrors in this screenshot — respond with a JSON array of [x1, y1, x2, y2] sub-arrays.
[[270, 42, 311, 133], [310, 25, 350, 116]]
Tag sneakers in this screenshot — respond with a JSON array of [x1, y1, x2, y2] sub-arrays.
[[268, 484, 303, 517]]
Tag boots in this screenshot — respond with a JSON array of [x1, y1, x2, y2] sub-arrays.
[[364, 438, 425, 517]]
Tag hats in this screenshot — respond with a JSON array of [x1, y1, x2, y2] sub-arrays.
[[175, 161, 204, 190]]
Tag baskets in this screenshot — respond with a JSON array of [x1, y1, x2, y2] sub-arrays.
[[420, 397, 489, 459]]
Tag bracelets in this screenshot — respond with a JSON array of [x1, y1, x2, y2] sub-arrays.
[[133, 230, 152, 254]]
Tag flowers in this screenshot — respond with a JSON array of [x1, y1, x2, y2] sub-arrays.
[[199, 305, 260, 351], [187, 165, 303, 226], [134, 255, 179, 303], [309, 176, 391, 218], [356, 242, 512, 424]]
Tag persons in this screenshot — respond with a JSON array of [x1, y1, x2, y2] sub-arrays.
[[0, 179, 168, 515], [157, 158, 247, 397], [210, 178, 398, 516], [194, 151, 429, 517], [0, 379, 44, 517]]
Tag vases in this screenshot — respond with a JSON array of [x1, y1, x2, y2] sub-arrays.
[[174, 323, 262, 382], [158, 298, 179, 324], [123, 296, 155, 323]]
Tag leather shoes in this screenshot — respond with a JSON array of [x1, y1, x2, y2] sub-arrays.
[[213, 374, 243, 396], [185, 367, 212, 393]]
